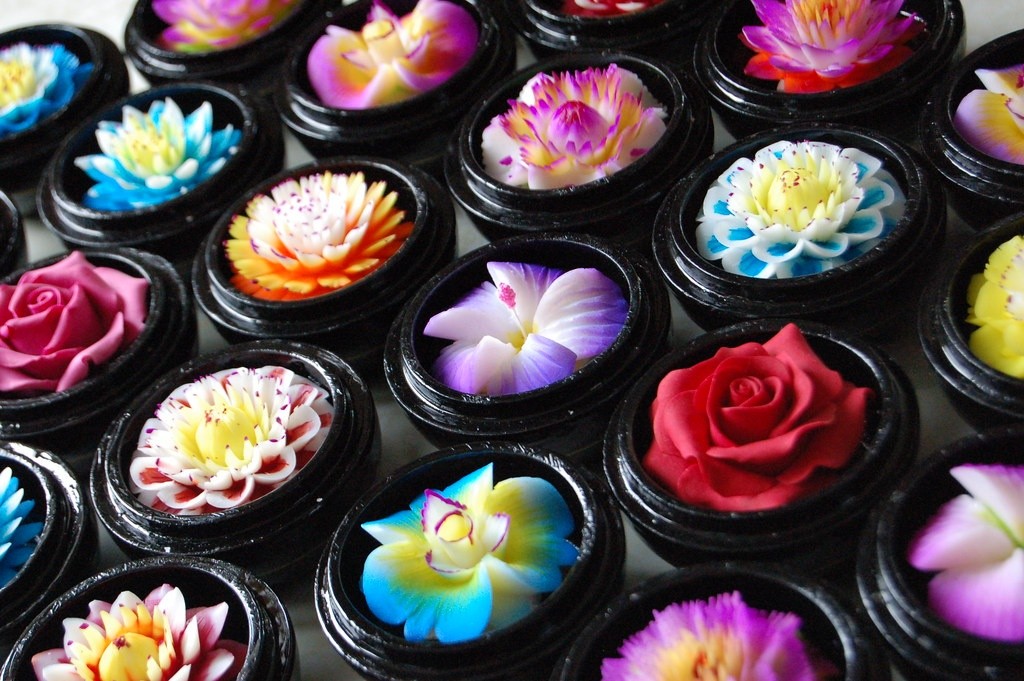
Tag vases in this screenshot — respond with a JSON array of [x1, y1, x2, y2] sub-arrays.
[[0, 0, 1024, 681]]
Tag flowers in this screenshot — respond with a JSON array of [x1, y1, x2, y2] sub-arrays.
[[361, 462, 581, 643], [966, 235, 1024, 381], [308, 0, 478, 107], [905, 462, 1023, 644], [0, 466, 43, 589], [481, 64, 667, 189], [1, 249, 148, 393], [953, 63, 1024, 164], [424, 262, 629, 395], [639, 322, 876, 511], [738, 0, 926, 94], [31, 584, 248, 680], [601, 589, 845, 681], [697, 138, 907, 279], [153, 0, 304, 53], [74, 97, 244, 213], [129, 364, 334, 514], [223, 170, 416, 300], [558, 0, 664, 17], [1, 41, 96, 137]]
